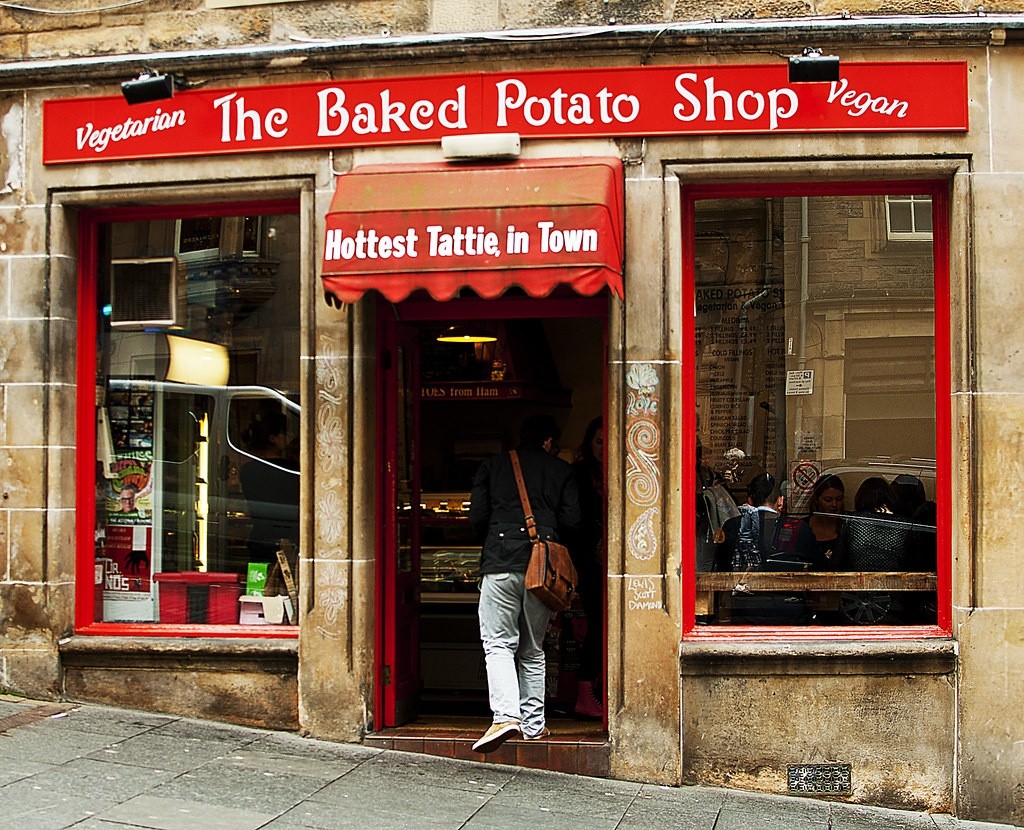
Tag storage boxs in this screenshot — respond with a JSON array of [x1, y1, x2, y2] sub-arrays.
[[153, 571, 239, 624], [245, 562, 270, 597]]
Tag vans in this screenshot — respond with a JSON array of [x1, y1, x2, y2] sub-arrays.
[[93, 376, 301, 583]]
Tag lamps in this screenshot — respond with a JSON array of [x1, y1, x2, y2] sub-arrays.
[[768, 46, 839, 83], [436, 320, 498, 343], [118, 59, 209, 106]]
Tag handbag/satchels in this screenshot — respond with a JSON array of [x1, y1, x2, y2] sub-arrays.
[[525, 541, 578, 612]]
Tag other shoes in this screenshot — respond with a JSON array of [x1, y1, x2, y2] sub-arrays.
[[523, 727, 550, 740], [472, 722, 520, 754]]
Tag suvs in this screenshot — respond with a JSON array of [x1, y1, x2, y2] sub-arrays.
[[766, 454, 936, 625]]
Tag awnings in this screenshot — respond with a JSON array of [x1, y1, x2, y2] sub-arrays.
[[319, 157, 627, 311]]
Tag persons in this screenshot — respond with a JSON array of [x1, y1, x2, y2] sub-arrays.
[[471, 404, 602, 756], [711, 470, 937, 627], [237, 406, 299, 564]]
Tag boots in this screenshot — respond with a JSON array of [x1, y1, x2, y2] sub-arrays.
[[575, 681, 602, 721]]
